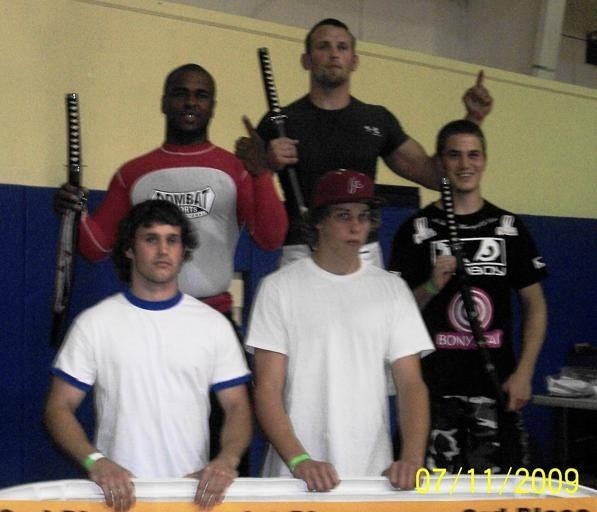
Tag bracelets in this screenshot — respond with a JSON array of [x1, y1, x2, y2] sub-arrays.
[[287, 453, 311, 471], [422, 279, 440, 297], [82, 450, 105, 473]]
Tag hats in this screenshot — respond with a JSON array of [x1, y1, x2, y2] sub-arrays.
[[311, 169, 389, 208]]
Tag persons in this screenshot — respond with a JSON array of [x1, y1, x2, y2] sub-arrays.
[[252, 17, 495, 268], [242, 167, 438, 493], [38, 197, 256, 512], [389, 118, 551, 478], [53, 62, 288, 480]]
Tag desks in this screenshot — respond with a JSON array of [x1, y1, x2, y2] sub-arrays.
[[530, 388, 597, 488]]
